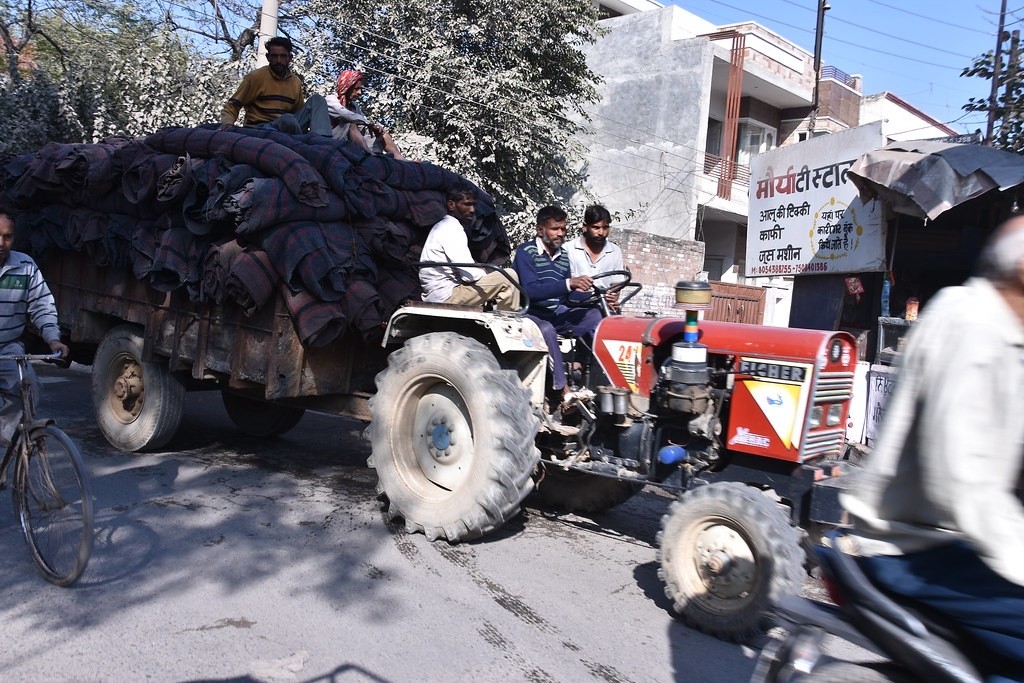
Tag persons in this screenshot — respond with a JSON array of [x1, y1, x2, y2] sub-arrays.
[[418, 181, 523, 313], [838, 214, 1024, 683], [324, 69, 412, 162], [513, 205, 621, 414], [220, 36, 334, 137], [0, 211, 70, 455], [560, 205, 624, 315]]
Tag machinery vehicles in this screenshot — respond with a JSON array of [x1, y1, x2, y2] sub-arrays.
[[48, 251, 862, 649]]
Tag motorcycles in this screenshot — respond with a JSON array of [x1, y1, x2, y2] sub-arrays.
[[766, 536, 1024, 683]]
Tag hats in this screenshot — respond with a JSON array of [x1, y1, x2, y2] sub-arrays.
[[336, 69, 363, 109]]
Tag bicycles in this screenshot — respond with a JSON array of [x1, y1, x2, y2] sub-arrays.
[[0, 347, 99, 585]]
